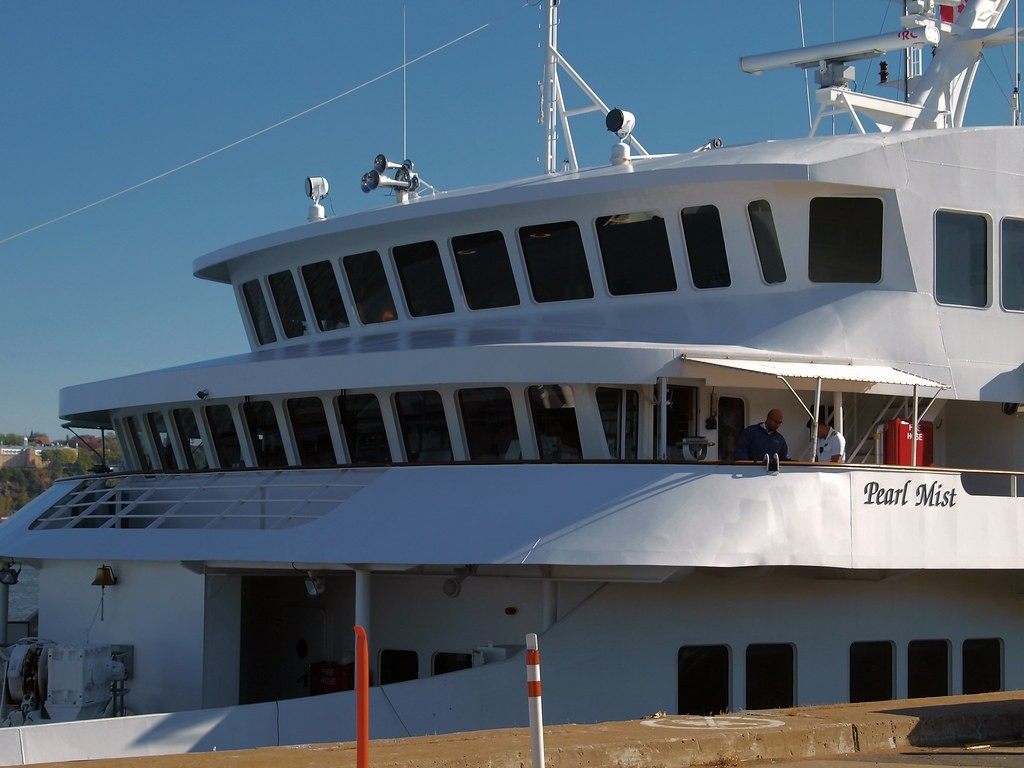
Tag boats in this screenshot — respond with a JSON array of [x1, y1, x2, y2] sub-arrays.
[[0, 0, 1024, 754]]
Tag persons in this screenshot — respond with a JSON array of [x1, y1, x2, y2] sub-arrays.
[[806, 416, 846, 463], [736, 409, 788, 461]]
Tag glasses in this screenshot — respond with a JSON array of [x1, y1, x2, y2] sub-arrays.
[[770, 416, 784, 425]]
